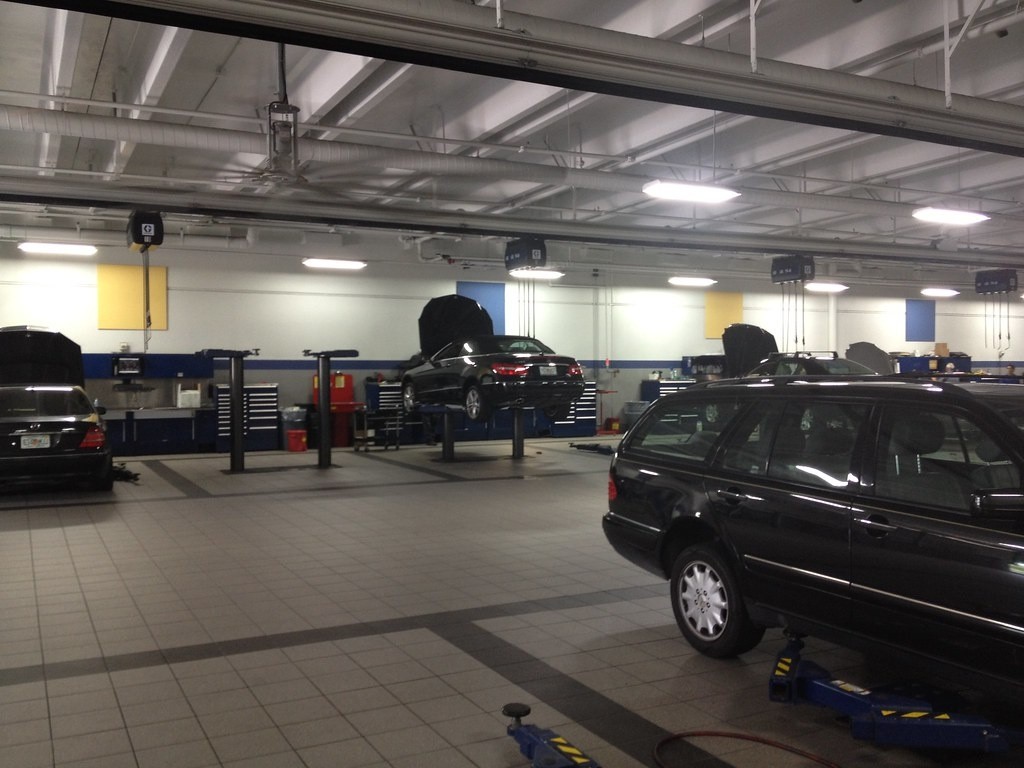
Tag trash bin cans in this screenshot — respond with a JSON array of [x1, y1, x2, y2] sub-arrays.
[[278, 409, 307, 451]]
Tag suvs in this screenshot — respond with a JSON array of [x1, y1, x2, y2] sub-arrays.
[[601, 373, 1024, 705]]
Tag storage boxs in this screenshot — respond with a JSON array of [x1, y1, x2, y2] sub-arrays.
[[935, 343, 951, 356], [177, 383, 201, 407]]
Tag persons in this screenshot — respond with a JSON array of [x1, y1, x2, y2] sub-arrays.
[[1000, 365, 1020, 384], [931, 362, 961, 384]]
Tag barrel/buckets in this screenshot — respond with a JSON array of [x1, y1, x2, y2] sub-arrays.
[[607, 417, 620, 433], [286, 430, 307, 452]]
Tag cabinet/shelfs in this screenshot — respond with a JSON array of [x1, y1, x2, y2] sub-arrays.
[[353, 404, 404, 452]]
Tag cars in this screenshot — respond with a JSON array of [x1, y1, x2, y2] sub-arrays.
[[696, 323, 883, 434], [400, 294, 584, 421], [844, 342, 984, 441], [0, 325, 114, 495]]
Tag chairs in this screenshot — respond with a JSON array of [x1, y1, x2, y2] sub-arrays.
[[883, 415, 966, 507], [972, 439, 1021, 489], [763, 425, 853, 487]]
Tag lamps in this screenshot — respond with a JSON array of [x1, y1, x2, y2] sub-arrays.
[[804, 282, 849, 292], [668, 277, 718, 288], [642, 176, 741, 203], [911, 204, 992, 225], [302, 257, 368, 270], [510, 269, 565, 281]]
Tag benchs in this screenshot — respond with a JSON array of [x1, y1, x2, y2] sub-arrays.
[[643, 431, 761, 470]]
